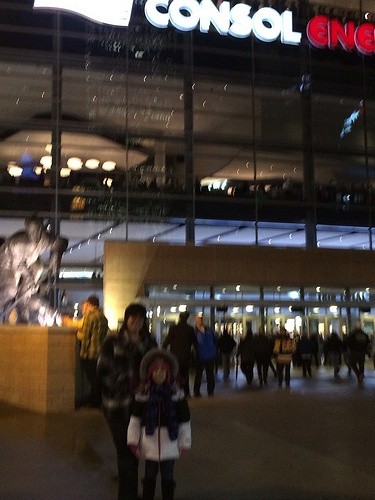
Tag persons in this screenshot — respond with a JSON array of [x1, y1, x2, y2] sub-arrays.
[[127, 350, 191, 500], [63, 295, 375, 410], [96, 302, 181, 500]]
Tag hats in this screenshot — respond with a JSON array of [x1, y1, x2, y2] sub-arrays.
[[147, 359, 171, 386]]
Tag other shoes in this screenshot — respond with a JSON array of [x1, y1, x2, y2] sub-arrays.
[[279, 383, 282, 386], [358, 373, 363, 384], [286, 383, 290, 387], [194, 392, 200, 397], [264, 379, 267, 384], [260, 379, 263, 384], [208, 391, 213, 395]]
